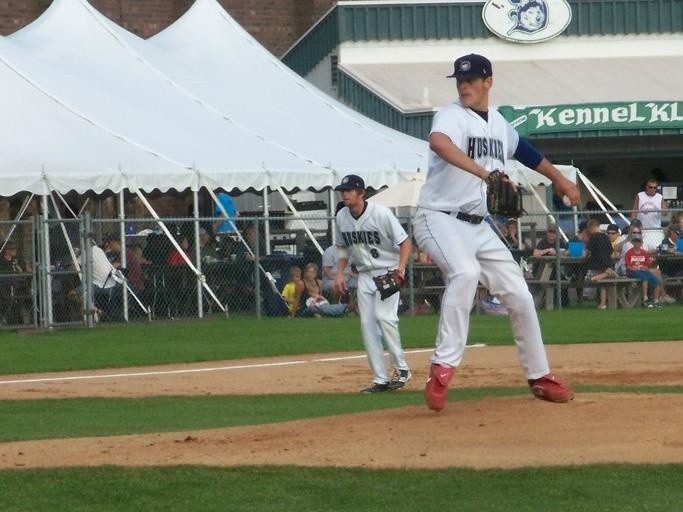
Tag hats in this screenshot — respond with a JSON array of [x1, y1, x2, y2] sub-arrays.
[[334, 174, 365, 193], [445, 53, 492, 81], [630, 233, 642, 242]]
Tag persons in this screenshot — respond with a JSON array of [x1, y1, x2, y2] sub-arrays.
[[414, 54, 580, 410], [335, 175, 411, 394]]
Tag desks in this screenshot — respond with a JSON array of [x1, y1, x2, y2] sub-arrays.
[[0, 254, 294, 325]]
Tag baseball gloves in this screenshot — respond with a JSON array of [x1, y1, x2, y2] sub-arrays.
[[372, 269, 405, 300], [486, 170, 522, 217]]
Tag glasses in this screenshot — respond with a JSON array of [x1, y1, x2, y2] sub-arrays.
[[647, 184, 657, 190]]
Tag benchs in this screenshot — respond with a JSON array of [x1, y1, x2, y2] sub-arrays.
[[400, 250, 683, 316]]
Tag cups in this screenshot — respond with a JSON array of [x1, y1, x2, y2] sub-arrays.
[[230, 254, 236, 261]]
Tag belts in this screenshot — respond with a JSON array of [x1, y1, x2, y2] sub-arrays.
[[439, 207, 487, 224]]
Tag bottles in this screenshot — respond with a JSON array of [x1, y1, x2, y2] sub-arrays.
[[660, 240, 668, 254]]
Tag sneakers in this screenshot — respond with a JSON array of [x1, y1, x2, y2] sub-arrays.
[[424, 363, 456, 412], [361, 381, 389, 393], [527, 374, 574, 403], [599, 267, 677, 309], [387, 369, 414, 391]]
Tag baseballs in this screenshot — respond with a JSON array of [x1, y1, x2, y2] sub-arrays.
[[562, 194, 573, 207]]
